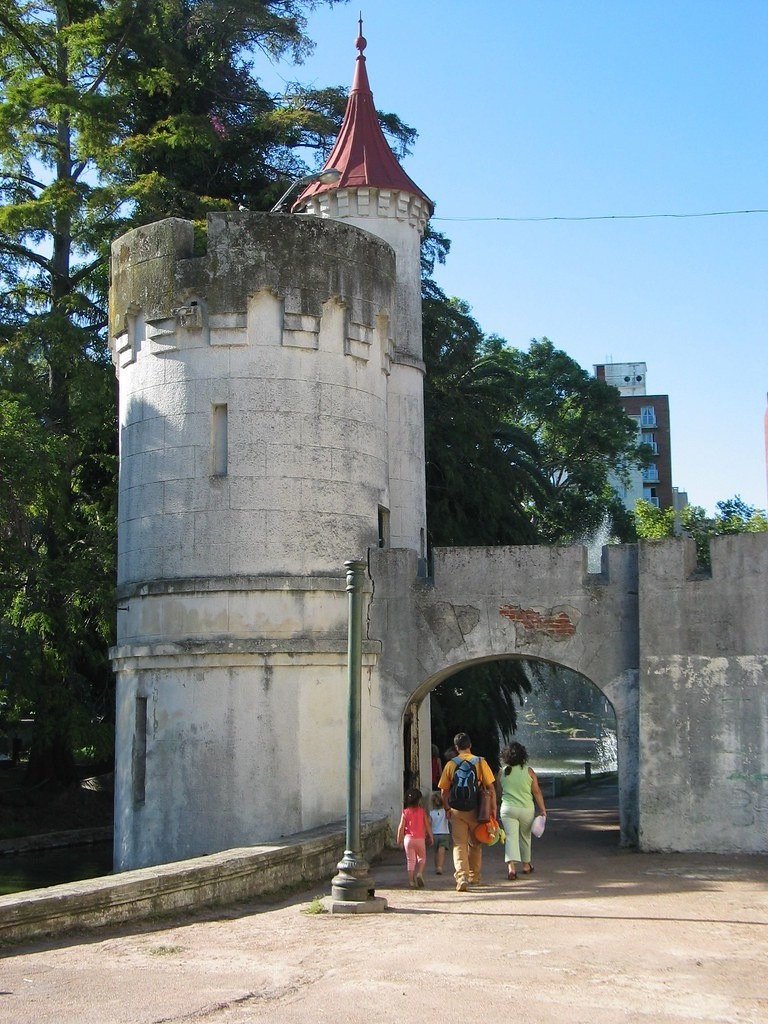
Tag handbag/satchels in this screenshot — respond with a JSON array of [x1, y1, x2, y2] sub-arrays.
[[478, 757, 493, 824]]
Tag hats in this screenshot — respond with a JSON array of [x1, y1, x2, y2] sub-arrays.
[[530, 816, 546, 838], [474, 815, 506, 847]]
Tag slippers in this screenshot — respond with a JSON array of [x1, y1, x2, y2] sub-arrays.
[[523, 863, 534, 874], [508, 871, 517, 879]]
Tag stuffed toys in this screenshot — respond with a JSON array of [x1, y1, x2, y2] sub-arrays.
[[474, 815, 506, 847]]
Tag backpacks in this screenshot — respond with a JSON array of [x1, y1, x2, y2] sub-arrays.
[[447, 756, 484, 813]]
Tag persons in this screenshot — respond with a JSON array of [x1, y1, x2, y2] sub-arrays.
[[431, 744, 442, 791], [428, 792, 451, 875], [438, 732, 497, 892], [496, 741, 547, 881], [396, 788, 434, 888]]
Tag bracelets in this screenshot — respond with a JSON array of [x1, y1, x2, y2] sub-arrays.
[[445, 807, 450, 812]]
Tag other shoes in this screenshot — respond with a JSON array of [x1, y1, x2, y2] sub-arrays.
[[436, 866, 443, 875], [456, 876, 468, 891], [468, 877, 480, 884], [409, 880, 416, 889], [416, 874, 425, 888]]
